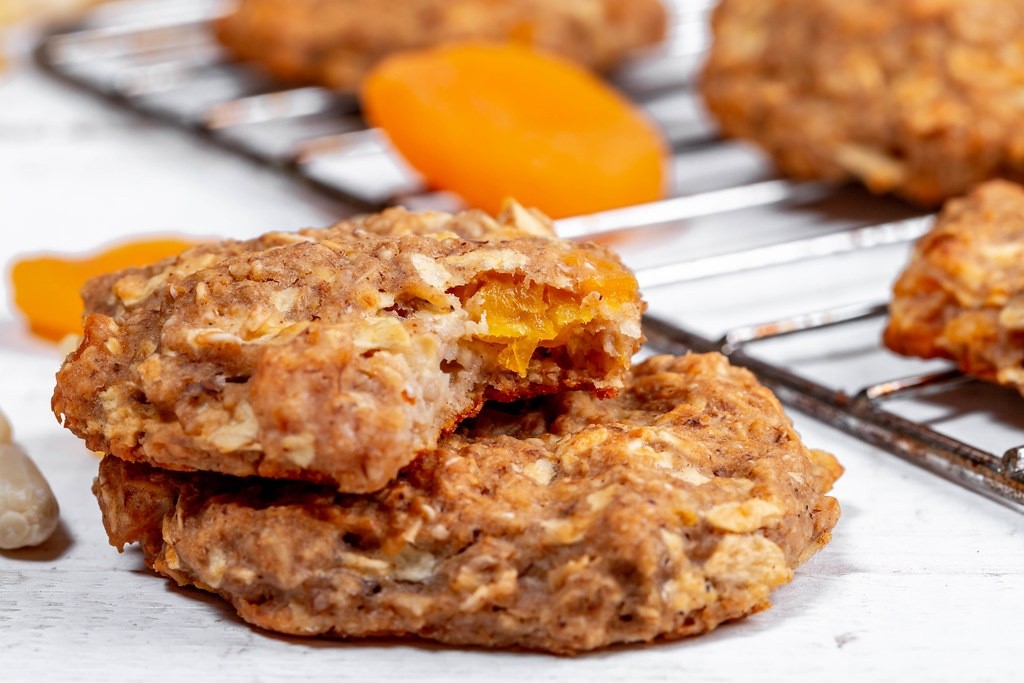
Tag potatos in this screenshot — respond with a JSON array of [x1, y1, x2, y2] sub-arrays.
[[11, 235, 207, 342], [359, 40, 669, 221]]
